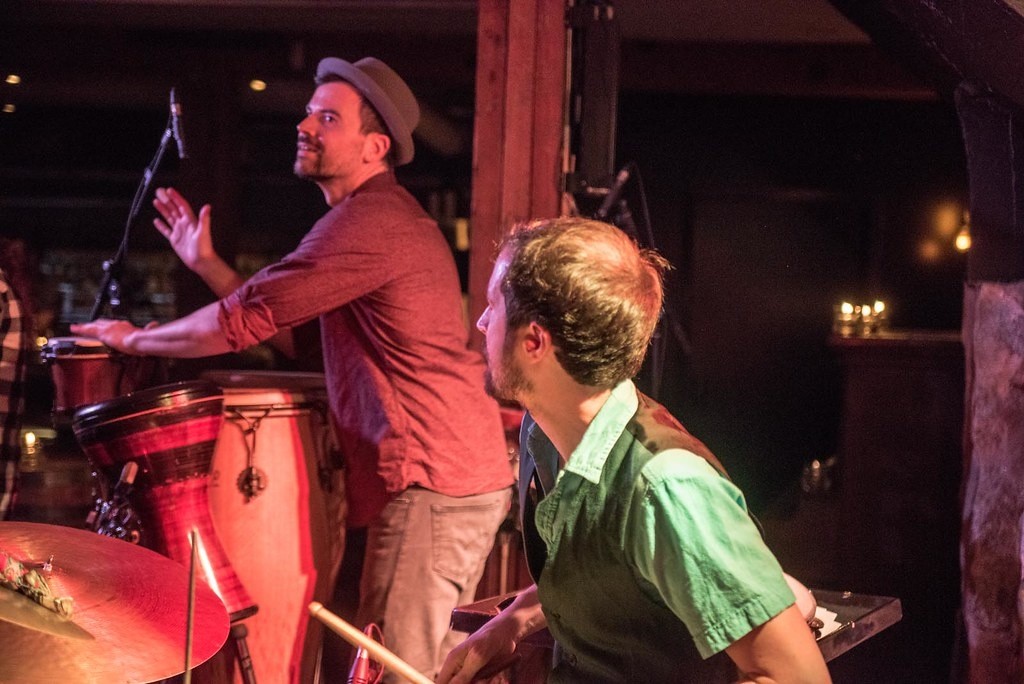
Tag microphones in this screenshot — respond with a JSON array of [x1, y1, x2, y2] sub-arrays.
[[169, 85, 187, 158], [597, 163, 632, 217]]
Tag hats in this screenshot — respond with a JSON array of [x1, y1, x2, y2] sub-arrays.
[[317, 57, 420, 166]]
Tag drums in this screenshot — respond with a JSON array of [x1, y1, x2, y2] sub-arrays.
[[214, 371, 340, 684], [72, 375, 259, 625], [40, 337, 141, 429]]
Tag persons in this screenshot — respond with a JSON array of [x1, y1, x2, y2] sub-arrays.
[[438, 216, 833, 684], [0, 201, 54, 524], [67, 57, 513, 684]]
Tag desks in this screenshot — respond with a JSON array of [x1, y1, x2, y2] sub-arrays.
[[450, 585, 904, 684], [822, 320, 965, 597]]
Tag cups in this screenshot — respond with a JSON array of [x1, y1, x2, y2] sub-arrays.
[[857, 314, 883, 338], [833, 312, 856, 338]]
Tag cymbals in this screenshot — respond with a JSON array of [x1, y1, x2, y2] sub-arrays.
[[0, 582, 95, 642], [0, 520, 231, 684]]
